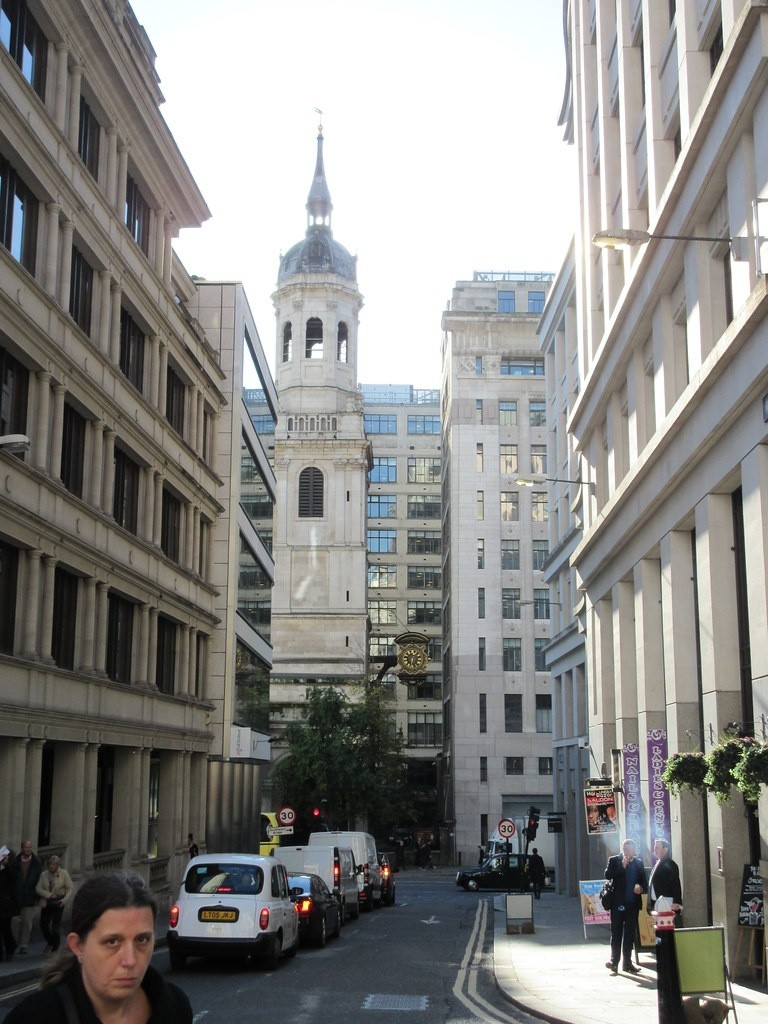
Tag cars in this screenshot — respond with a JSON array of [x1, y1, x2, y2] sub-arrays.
[[286, 871, 343, 948], [167, 852, 304, 972]]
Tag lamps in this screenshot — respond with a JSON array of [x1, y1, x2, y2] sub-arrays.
[[517, 600, 563, 612], [585, 776, 611, 786], [0, 433, 32, 454], [514, 475, 595, 494], [592, 229, 740, 265]]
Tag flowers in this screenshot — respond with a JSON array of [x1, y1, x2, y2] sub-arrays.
[[660, 736, 768, 807]]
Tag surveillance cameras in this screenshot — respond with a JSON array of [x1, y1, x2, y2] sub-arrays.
[[432, 762, 435, 765], [579, 737, 584, 750]]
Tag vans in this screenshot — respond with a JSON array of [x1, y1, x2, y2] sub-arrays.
[[307, 831, 387, 912], [377, 852, 400, 907], [274, 845, 366, 927]]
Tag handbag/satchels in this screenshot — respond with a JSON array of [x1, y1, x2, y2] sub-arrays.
[[545, 875, 551, 886], [600, 882, 615, 910]]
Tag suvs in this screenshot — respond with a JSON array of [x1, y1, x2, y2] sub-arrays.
[[456, 853, 551, 892]]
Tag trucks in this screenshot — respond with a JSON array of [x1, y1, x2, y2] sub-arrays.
[[481, 815, 557, 889]]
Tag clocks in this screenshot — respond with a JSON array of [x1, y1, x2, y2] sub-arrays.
[[396, 639, 430, 676]]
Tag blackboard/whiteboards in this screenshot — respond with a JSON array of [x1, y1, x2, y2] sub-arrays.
[[737, 864, 765, 929]]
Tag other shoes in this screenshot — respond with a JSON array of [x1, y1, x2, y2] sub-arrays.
[[20, 949, 27, 954], [5, 945, 18, 962], [607, 962, 617, 972], [623, 965, 639, 972], [44, 945, 51, 953]]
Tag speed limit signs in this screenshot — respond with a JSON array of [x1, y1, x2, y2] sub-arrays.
[[276, 806, 297, 826], [498, 818, 515, 838]]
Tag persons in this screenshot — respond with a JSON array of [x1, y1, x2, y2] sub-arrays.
[[587, 805, 617, 833], [188, 833, 198, 859], [530, 848, 549, 899], [5, 868, 193, 1024], [646, 840, 683, 928], [415, 838, 436, 870], [396, 841, 405, 870], [0, 840, 73, 963], [478, 846, 484, 864], [605, 839, 649, 973]]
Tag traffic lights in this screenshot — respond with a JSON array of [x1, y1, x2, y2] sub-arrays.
[[527, 807, 540, 840], [312, 808, 322, 832]]
